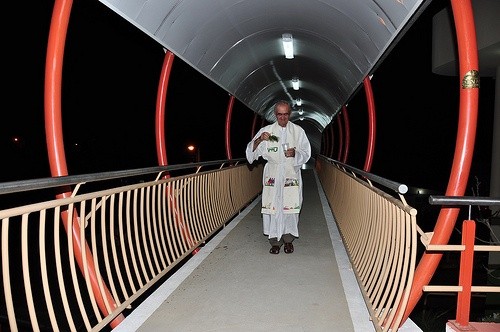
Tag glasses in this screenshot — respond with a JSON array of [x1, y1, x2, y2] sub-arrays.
[[267, 132, 278, 141], [276, 112, 290, 116]]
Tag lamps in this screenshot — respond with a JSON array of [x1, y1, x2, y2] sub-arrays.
[[292, 77, 299, 90], [297, 98, 304, 120], [282, 33, 294, 59]]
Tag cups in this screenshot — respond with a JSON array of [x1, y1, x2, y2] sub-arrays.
[[282, 143, 289, 156]]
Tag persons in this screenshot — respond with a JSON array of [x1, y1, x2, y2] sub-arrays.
[[246, 100, 311, 254]]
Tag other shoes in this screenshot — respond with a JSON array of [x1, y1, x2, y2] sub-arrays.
[[270, 245, 280, 254], [284, 242, 294, 254]]
